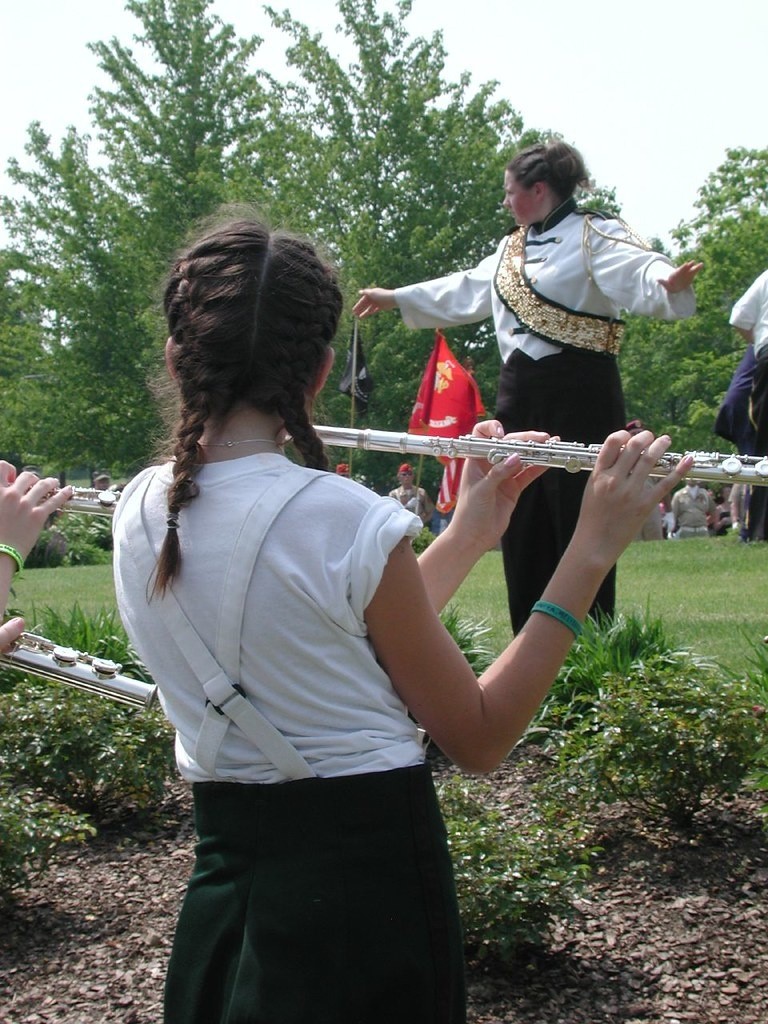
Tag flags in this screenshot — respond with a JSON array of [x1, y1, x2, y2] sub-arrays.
[[339, 328, 375, 419], [408, 335, 486, 514]]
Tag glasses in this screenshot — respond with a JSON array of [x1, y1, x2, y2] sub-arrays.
[[401, 472, 413, 476]]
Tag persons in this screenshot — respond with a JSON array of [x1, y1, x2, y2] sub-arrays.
[[352, 142, 703, 639], [729, 269, 768, 544], [336, 462, 349, 478], [714, 344, 756, 542], [389, 463, 434, 524], [89, 469, 125, 493], [0, 459, 72, 651], [24, 464, 63, 530], [110, 220, 695, 1024], [626, 419, 745, 541]]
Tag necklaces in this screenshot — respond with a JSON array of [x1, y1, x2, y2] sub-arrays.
[[198, 439, 277, 446]]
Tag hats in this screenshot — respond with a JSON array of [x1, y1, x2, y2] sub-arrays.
[[92, 471, 112, 482], [23, 465, 40, 476], [399, 464, 413, 472], [336, 464, 350, 474]]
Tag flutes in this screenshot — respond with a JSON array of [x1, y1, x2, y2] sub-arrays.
[[288, 420, 768, 488], [0, 631, 159, 716], [8, 478, 121, 517]]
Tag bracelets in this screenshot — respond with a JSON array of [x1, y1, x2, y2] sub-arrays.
[[0, 544, 24, 583], [530, 600, 581, 640]]
